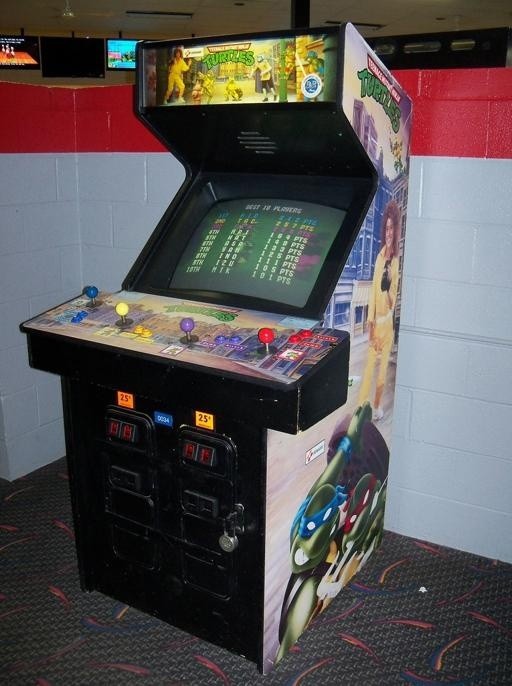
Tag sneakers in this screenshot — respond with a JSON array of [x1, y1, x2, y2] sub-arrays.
[[372, 405, 384, 421]]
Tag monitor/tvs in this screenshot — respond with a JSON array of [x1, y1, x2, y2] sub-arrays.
[[106, 38, 141, 71], [40, 36, 105, 78], [169, 198, 346, 308], [0, 35, 40, 70]]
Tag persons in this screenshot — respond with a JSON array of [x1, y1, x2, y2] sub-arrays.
[[163, 49, 192, 105], [358, 202, 401, 420], [248, 54, 277, 102]]
